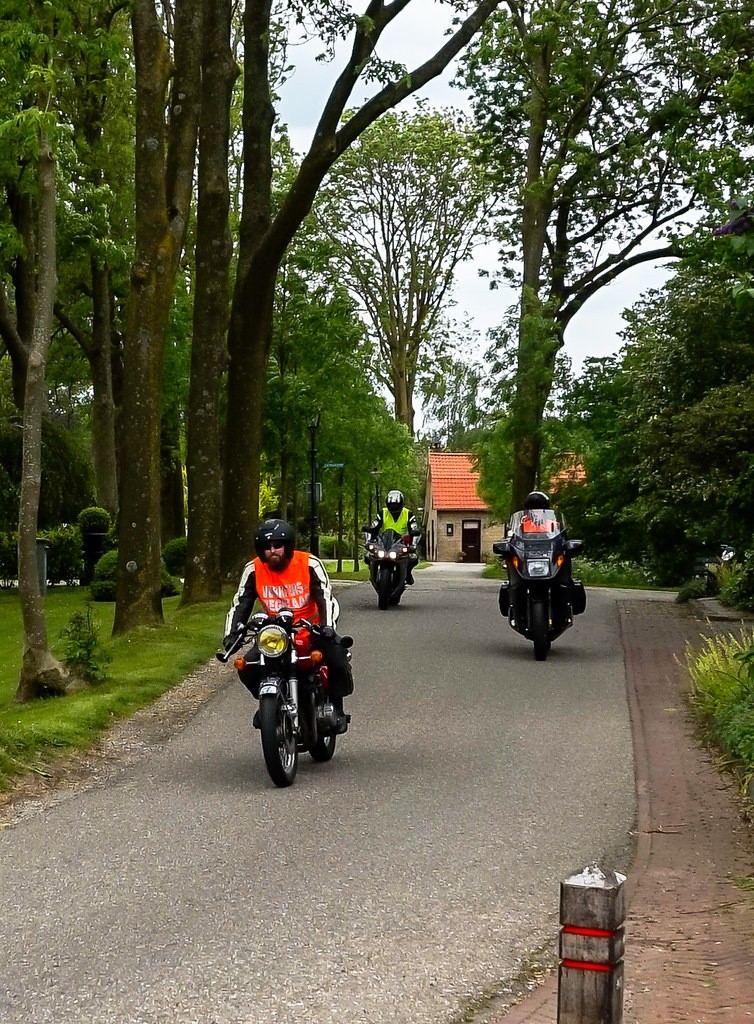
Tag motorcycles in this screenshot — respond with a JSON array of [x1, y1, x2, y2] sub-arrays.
[[217, 607, 353, 787], [359, 526, 421, 610], [493, 508, 586, 661]]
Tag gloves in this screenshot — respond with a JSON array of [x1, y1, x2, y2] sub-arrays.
[[322, 626, 337, 642], [223, 635, 241, 655]]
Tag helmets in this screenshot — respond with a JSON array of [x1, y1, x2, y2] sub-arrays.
[[254, 519, 296, 562], [525, 491, 550, 509], [386, 490, 404, 514]]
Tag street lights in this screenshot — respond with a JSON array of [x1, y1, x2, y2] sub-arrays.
[[304, 410, 321, 555]]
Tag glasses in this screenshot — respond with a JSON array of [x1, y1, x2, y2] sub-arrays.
[[264, 542, 284, 550]]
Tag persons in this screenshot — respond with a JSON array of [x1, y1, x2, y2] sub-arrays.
[[222, 519, 348, 727], [494, 491, 584, 613], [362, 490, 420, 585]]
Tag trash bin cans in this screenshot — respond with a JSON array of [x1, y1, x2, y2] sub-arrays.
[[35, 538, 50, 597]]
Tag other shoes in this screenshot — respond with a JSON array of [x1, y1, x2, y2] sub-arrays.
[[333, 714, 347, 734], [406, 573, 414, 585]]
